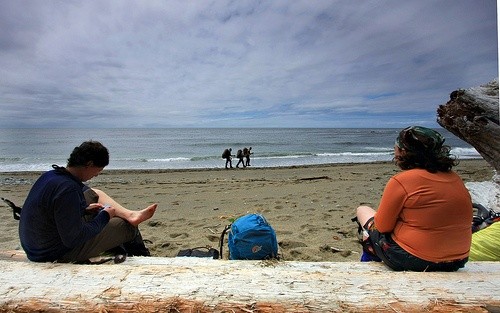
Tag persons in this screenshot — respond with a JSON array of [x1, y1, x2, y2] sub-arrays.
[[19, 139, 158, 265], [356, 125, 474, 273], [245, 147, 254, 166], [235, 149, 246, 168], [221, 148, 235, 169]]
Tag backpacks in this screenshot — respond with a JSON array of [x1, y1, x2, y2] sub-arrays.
[[220, 213, 278, 261]]
